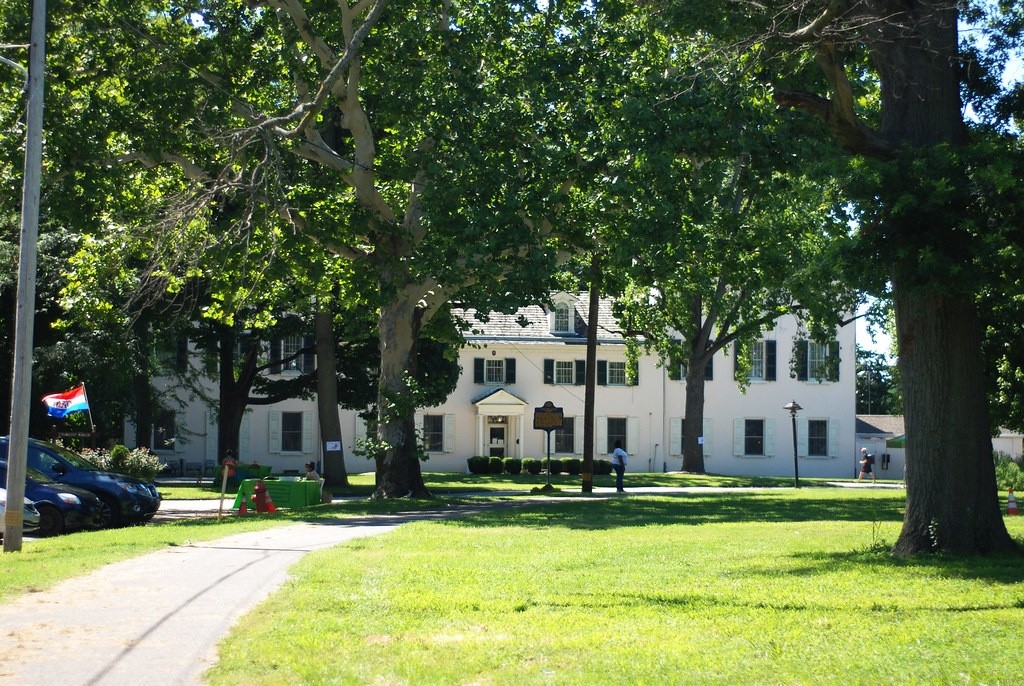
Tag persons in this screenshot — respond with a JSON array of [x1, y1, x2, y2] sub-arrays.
[[612, 439, 629, 493], [853, 448, 875, 484], [304, 461, 321, 480]]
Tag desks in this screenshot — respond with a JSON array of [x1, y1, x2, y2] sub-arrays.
[[239, 478, 321, 508]]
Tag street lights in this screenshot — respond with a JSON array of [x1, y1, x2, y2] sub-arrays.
[[782, 400, 803, 488]]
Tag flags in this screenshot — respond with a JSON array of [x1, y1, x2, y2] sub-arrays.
[[42, 385, 88, 418]]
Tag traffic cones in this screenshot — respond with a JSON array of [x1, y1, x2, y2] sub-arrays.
[[1007, 488, 1018, 516], [265, 489, 274, 514], [239, 492, 248, 516]]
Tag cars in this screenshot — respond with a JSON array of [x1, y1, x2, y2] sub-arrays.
[[0, 458, 103, 540], [0, 435, 161, 527], [0, 488, 40, 539]]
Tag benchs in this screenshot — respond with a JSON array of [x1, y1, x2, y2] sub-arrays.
[[185, 461, 202, 476], [204, 462, 216, 475]]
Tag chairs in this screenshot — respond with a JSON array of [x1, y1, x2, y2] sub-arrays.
[[319, 477, 326, 503]]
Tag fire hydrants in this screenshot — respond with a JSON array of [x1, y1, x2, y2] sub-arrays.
[[251, 480, 266, 514]]
